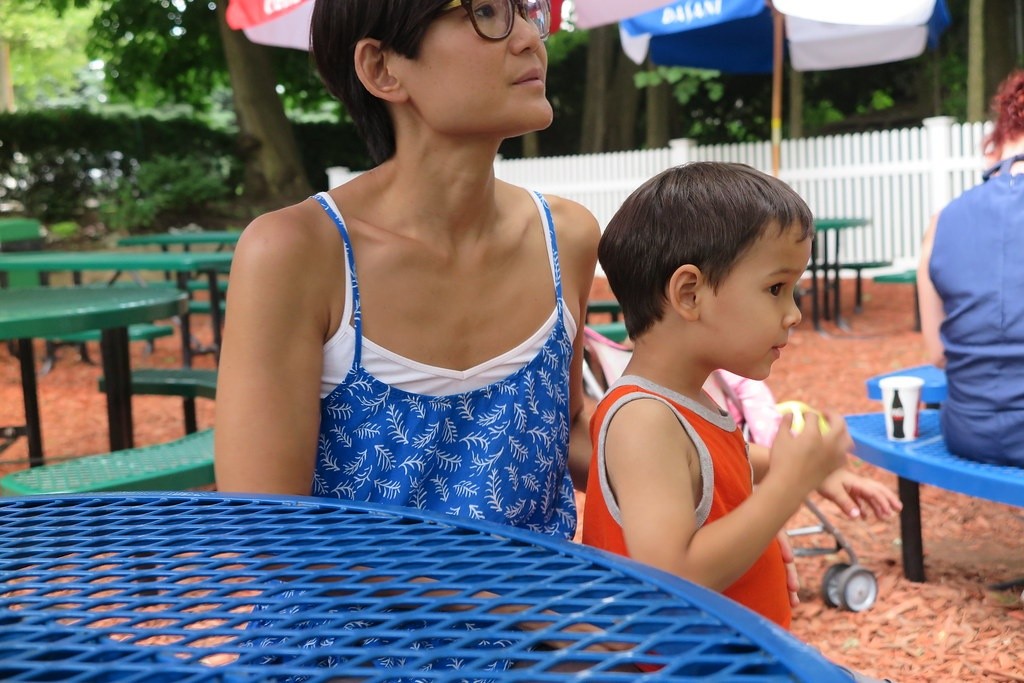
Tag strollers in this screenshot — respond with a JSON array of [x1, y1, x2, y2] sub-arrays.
[[579, 328, 881, 612]]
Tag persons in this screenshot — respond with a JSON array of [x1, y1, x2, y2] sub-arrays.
[[581, 161, 903, 673], [213, 0, 590, 683], [917, 69, 1024, 467]]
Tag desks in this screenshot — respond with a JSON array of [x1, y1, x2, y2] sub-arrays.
[[0, 251, 235, 431], [0, 478, 859, 681], [0, 285, 187, 464], [117, 233, 245, 279], [800, 218, 873, 335]]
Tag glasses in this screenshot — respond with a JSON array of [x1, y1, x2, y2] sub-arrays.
[[431, 0, 551, 40]]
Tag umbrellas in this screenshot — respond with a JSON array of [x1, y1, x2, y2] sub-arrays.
[[227, 0, 953, 180]]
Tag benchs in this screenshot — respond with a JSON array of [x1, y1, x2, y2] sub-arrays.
[[582, 300, 630, 355], [804, 259, 922, 335], [846, 364, 1024, 581], [0, 267, 252, 492]]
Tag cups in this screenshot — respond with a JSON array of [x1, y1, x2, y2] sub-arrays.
[[878, 377, 925, 442]]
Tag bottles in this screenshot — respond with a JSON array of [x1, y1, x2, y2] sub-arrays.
[[891, 389, 905, 438]]
